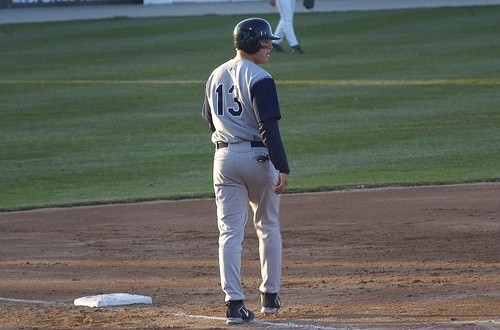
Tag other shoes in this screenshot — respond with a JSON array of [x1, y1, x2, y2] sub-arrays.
[[291, 45, 304, 54], [271, 43, 285, 52]]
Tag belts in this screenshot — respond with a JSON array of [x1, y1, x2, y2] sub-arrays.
[[217, 141, 266, 149]]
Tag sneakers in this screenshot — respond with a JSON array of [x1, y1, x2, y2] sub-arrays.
[[261, 292, 281, 314], [226, 303, 255, 324]]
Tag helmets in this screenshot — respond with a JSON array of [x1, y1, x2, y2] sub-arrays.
[[233, 18, 281, 48]]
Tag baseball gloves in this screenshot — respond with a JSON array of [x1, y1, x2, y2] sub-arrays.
[[304, 0, 314, 8]]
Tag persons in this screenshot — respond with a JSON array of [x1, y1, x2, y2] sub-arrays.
[[202, 18, 291, 323], [267, 0, 315, 54]]
